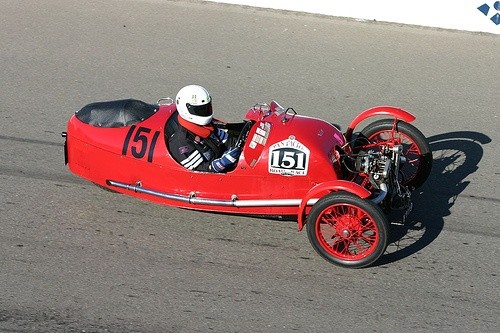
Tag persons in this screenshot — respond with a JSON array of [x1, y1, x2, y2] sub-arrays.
[[169, 84, 240, 173]]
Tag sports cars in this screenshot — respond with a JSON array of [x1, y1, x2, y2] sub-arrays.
[[61, 98, 433, 269]]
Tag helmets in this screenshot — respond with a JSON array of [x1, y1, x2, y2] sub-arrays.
[[175, 85, 213, 126]]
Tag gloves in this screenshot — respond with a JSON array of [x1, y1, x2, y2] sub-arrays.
[[212, 146, 240, 172], [209, 127, 228, 145]]
